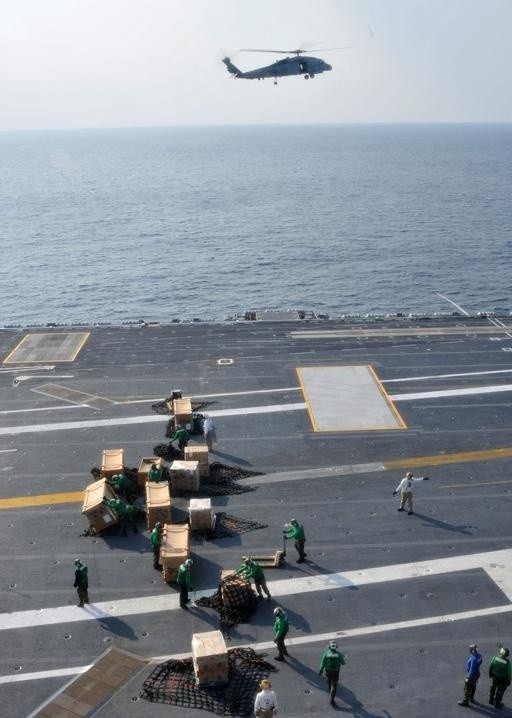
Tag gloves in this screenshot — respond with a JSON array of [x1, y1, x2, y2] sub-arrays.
[[273, 638, 277, 643], [318, 670, 323, 676], [73, 584, 78, 587], [282, 530, 287, 533], [283, 536, 288, 540], [423, 477, 429, 480], [392, 491, 397, 496]]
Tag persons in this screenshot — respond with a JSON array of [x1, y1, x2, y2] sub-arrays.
[[168, 424, 190, 450], [272, 606, 289, 662], [150, 522, 164, 570], [151, 523, 168, 537], [253, 679, 279, 718], [202, 417, 213, 452], [457, 644, 482, 707], [488, 646, 512, 709], [74, 558, 90, 608], [393, 472, 429, 515], [177, 557, 194, 611], [282, 518, 307, 563], [126, 504, 145, 520], [102, 496, 138, 537], [105, 473, 138, 503], [237, 558, 271, 602], [147, 464, 161, 483], [317, 640, 346, 705]]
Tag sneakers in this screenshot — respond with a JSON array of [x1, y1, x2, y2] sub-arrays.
[[180, 599, 191, 610], [154, 563, 163, 570], [408, 511, 413, 516], [397, 508, 403, 512], [77, 599, 89, 607], [119, 528, 138, 537], [127, 496, 138, 505], [256, 594, 271, 603]]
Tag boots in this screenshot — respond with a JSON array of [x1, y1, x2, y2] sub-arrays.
[[470, 695, 476, 704], [488, 695, 495, 704], [273, 647, 288, 662], [296, 551, 306, 564], [330, 694, 337, 705], [457, 697, 469, 706], [494, 699, 505, 709]]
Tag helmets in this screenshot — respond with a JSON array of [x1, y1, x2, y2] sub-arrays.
[[74, 559, 81, 566], [261, 679, 272, 688], [150, 464, 156, 469], [469, 644, 478, 653], [328, 640, 337, 650], [406, 471, 413, 476], [185, 559, 192, 567], [243, 557, 252, 565], [154, 522, 162, 528], [500, 647, 510, 657], [175, 424, 181, 430], [274, 607, 284, 615], [112, 474, 119, 481], [290, 519, 298, 526], [109, 499, 115, 504]]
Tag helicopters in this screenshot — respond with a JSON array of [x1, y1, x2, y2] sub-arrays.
[[223, 48, 332, 85]]
